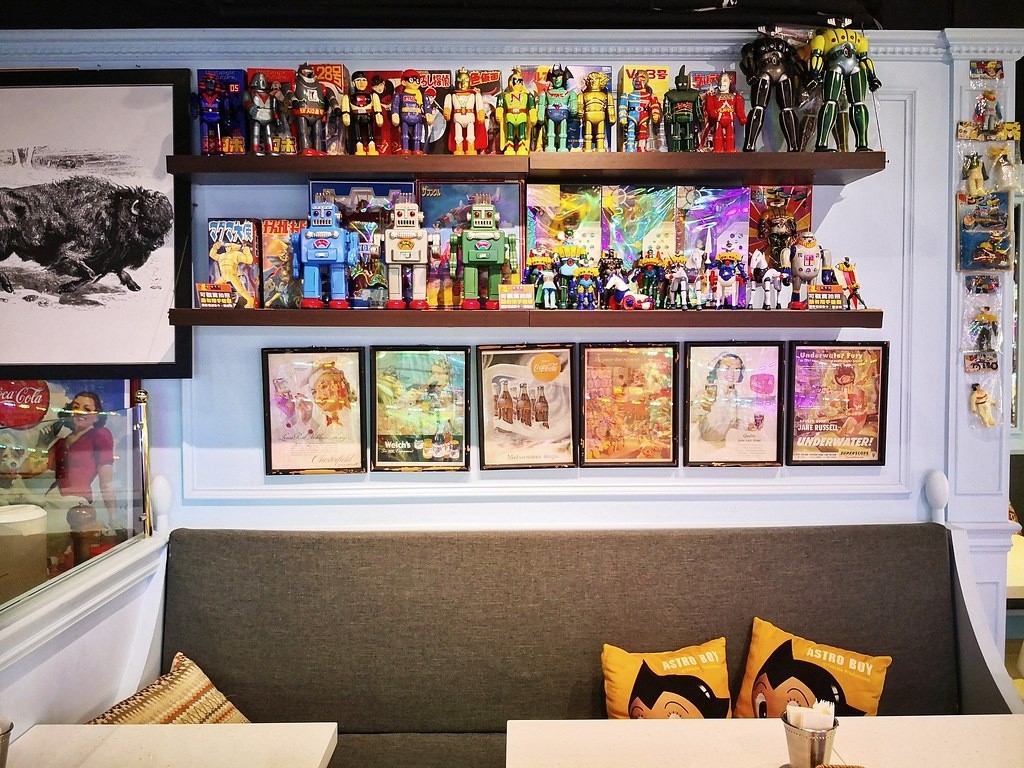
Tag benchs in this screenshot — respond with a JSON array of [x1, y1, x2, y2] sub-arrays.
[[161, 523, 955, 768]]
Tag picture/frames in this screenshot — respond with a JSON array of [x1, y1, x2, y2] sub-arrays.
[[0, 70, 193, 379], [786, 341, 890, 466], [261, 346, 367, 475], [370, 345, 472, 472], [579, 342, 679, 467], [476, 342, 578, 470], [683, 341, 786, 467]]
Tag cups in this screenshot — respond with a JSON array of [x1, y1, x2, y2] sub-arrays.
[[780, 709, 839, 768]]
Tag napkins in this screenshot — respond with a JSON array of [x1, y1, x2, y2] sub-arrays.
[[786, 698, 835, 729]]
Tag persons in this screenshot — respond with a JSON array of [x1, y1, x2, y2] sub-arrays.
[[740, 15, 882, 152], [975, 90, 1002, 134], [971, 383, 997, 427], [17, 392, 119, 531], [963, 153, 990, 197], [291, 194, 867, 310], [187, 64, 750, 155]]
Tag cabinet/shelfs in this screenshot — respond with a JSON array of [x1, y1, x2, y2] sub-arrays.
[[166, 152, 886, 327]]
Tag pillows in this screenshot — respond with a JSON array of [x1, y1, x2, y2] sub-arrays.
[[84, 652, 252, 725], [733, 617, 893, 718], [601, 637, 733, 720]]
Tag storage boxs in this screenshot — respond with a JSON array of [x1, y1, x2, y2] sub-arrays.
[[196, 64, 736, 308]]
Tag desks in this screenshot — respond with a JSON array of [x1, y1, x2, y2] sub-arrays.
[[5, 722, 339, 768], [506, 714, 1024, 768]]
